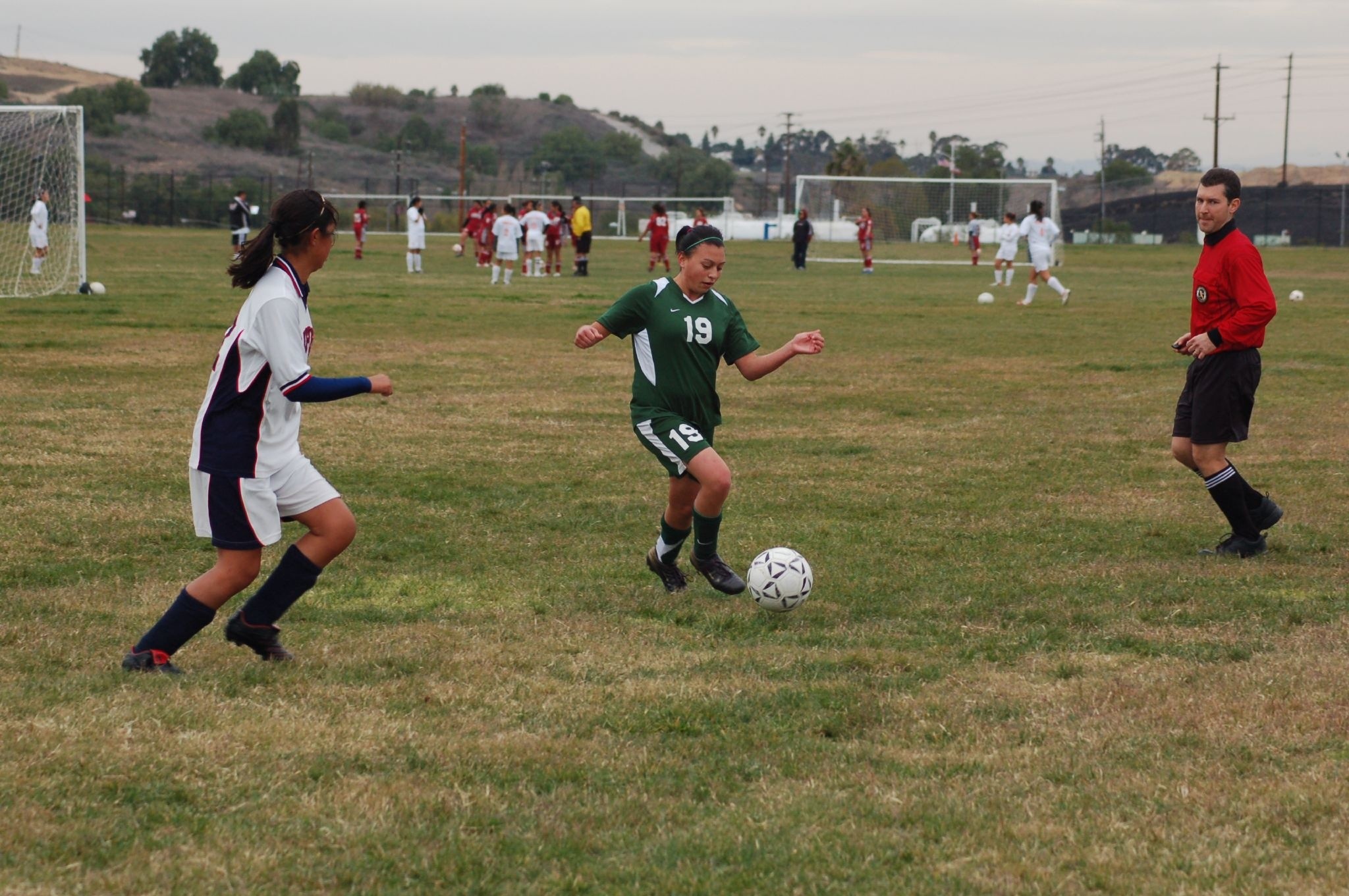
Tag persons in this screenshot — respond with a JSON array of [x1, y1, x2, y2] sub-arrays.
[[28, 187, 1073, 311], [1170, 168, 1282, 558], [120, 191, 398, 676], [571, 225, 824, 596]]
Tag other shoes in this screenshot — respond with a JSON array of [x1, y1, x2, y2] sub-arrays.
[[29, 269, 41, 275], [542, 273, 548, 277], [863, 268, 872, 274], [233, 254, 241, 259], [573, 271, 587, 275], [553, 272, 560, 277], [1016, 301, 1024, 305], [476, 263, 491, 267], [989, 281, 1003, 286], [1062, 287, 1071, 306]]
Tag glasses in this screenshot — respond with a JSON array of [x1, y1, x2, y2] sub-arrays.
[[316, 229, 336, 246]]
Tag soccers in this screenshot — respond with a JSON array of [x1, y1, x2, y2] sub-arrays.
[[978, 293, 994, 303], [1290, 290, 1303, 302], [89, 281, 106, 295], [452, 244, 461, 253], [747, 547, 813, 612]]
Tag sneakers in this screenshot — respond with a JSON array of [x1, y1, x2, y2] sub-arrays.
[[225, 611, 294, 662], [688, 551, 746, 595], [1198, 531, 1267, 558], [1245, 492, 1284, 530], [647, 548, 694, 592], [121, 647, 182, 674]]
